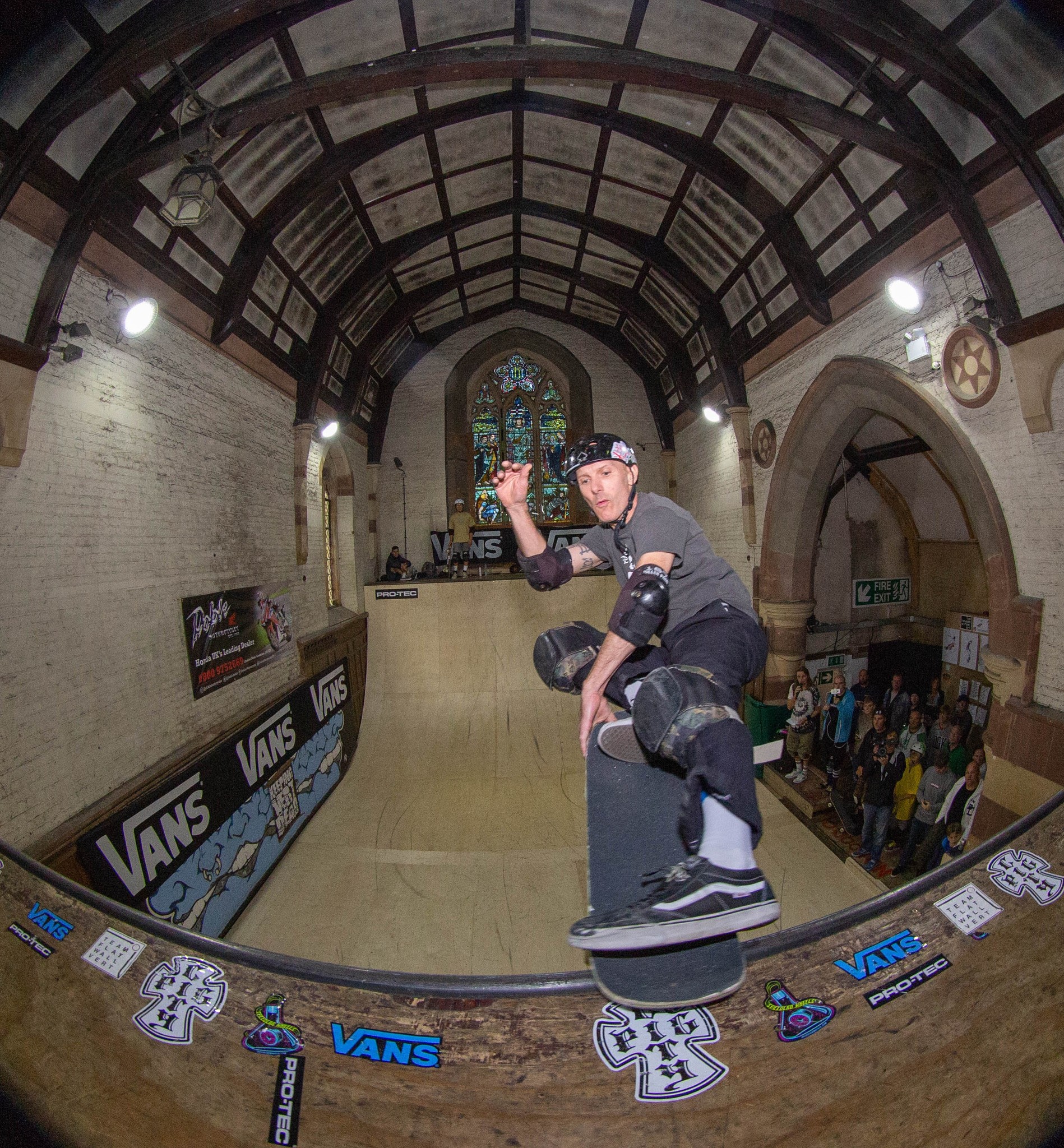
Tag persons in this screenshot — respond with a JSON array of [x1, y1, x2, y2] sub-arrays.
[[448, 499, 476, 582], [386, 546, 412, 581], [492, 431, 783, 950], [780, 669, 990, 881]]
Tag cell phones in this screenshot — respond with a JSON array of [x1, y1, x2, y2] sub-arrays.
[[831, 689, 840, 694]]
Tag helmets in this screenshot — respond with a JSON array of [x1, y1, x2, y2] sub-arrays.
[[564, 432, 638, 481], [909, 741, 926, 756], [454, 498, 465, 506]]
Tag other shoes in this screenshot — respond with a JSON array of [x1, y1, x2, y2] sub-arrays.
[[851, 848, 870, 857], [820, 779, 836, 796], [884, 841, 900, 852], [864, 858, 879, 871], [785, 770, 807, 784], [891, 867, 900, 878]]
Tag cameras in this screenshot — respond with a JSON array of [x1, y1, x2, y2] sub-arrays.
[[876, 745, 886, 758]]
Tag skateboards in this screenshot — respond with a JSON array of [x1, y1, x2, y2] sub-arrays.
[[827, 790, 862, 837], [444, 547, 451, 578], [582, 705, 752, 1010]]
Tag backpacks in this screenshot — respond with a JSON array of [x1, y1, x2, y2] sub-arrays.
[[422, 561, 438, 579]]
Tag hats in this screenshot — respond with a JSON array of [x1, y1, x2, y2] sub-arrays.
[[956, 694, 970, 702]]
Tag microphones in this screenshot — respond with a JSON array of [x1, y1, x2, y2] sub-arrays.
[[795, 683, 803, 700]]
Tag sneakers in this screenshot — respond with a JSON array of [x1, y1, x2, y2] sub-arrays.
[[462, 572, 468, 578], [568, 856, 781, 951], [596, 715, 654, 764], [400, 577, 412, 581], [452, 572, 457, 579]]
